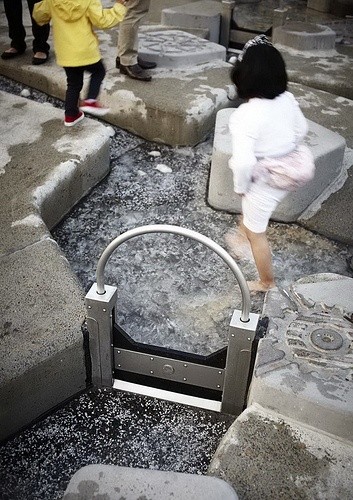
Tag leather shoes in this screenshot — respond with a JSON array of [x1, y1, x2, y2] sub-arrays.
[[115, 55, 156, 82]]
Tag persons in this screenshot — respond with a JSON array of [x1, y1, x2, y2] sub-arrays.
[[228, 34, 316, 291], [32, 0, 129, 126], [116, 0, 157, 81], [1, 0, 50, 65]]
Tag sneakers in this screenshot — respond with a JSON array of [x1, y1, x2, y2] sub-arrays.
[[0, 47, 26, 60], [30, 49, 50, 65], [64, 111, 85, 125], [80, 101, 112, 117]]
